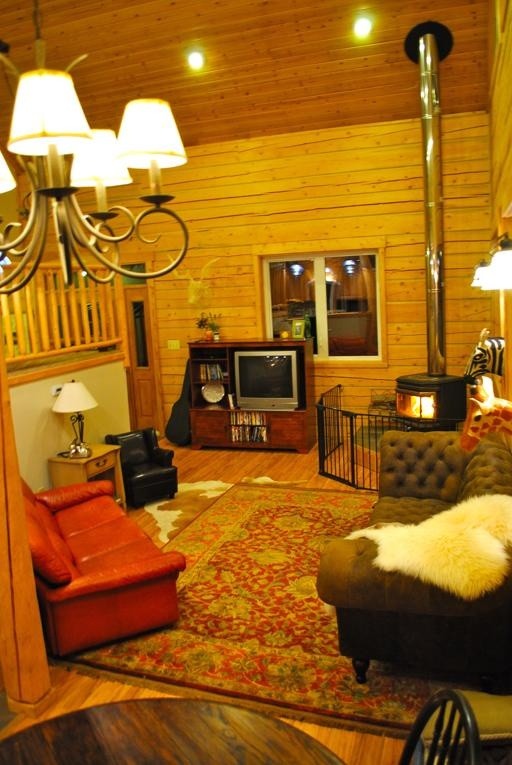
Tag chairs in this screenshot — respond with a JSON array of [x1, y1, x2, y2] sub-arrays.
[[328, 311, 372, 356], [105, 427, 178, 507], [397, 688, 478, 764]]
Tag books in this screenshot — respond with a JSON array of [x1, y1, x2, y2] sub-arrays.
[[200, 364, 223, 380], [230, 412, 267, 443]]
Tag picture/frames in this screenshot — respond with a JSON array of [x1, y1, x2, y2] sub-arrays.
[[292, 320, 305, 338]]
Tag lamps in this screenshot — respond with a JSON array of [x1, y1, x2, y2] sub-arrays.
[[51, 379, 98, 458], [0, 0, 191, 293], [469, 232, 512, 341]]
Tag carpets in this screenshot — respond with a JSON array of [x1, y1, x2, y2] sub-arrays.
[[47, 482, 432, 744]]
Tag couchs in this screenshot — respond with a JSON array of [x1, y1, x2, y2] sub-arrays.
[[316, 431, 511, 697], [16, 476, 186, 658]]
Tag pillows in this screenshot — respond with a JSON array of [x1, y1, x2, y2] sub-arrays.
[[421, 679, 511, 745]]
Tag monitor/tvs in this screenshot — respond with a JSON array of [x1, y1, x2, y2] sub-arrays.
[[234, 349, 300, 411]]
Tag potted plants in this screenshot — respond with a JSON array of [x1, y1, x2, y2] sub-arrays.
[[194, 312, 212, 340], [210, 321, 220, 342]]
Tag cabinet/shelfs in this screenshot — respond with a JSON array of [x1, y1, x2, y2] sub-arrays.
[[189, 339, 317, 456], [49, 443, 126, 510]]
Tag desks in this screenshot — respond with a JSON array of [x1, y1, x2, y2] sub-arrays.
[[1, 699, 346, 765]]
